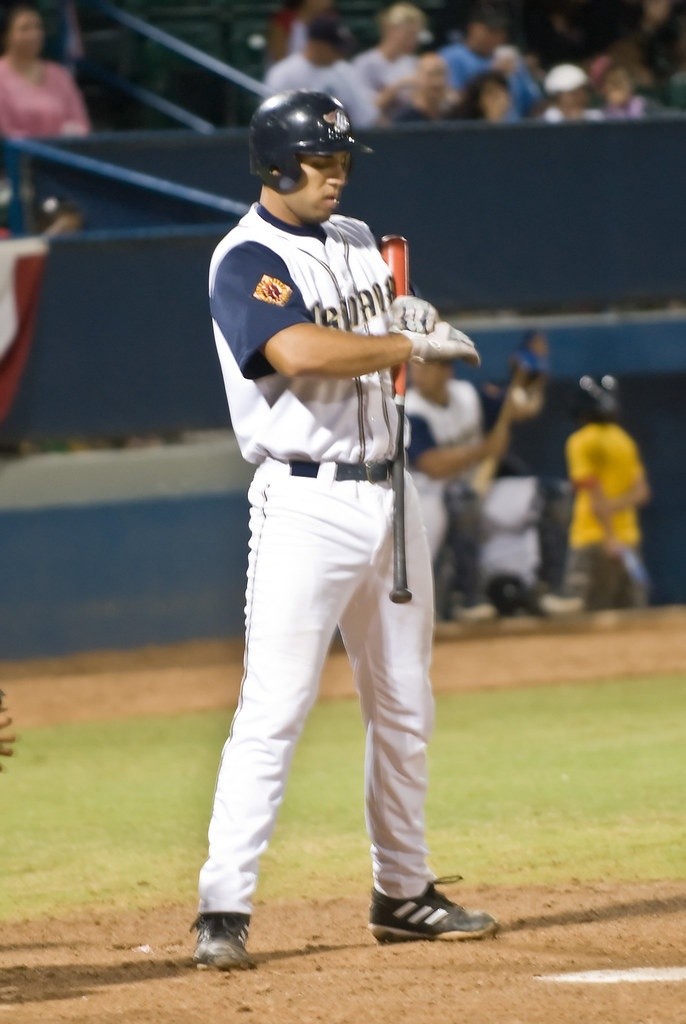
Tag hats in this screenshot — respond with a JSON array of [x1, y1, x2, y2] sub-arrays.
[[307, 15, 359, 51], [379, 5, 432, 43], [544, 64, 588, 95]]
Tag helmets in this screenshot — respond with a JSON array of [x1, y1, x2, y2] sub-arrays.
[[249, 89, 373, 194]]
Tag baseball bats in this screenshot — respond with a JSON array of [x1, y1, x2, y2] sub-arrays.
[[378, 233, 414, 606]]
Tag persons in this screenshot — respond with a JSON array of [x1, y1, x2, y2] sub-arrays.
[[0, 181, 87, 425], [190, 89, 499, 971], [259, 2, 686, 129], [2, 1, 96, 142], [402, 355, 652, 638]]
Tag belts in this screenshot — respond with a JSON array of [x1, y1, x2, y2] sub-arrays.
[[289, 460, 393, 484]]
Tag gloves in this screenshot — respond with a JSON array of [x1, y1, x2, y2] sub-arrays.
[[389, 296, 480, 366]]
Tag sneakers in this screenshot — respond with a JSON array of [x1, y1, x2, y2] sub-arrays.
[[188, 912, 254, 971], [369, 876, 498, 946]]
